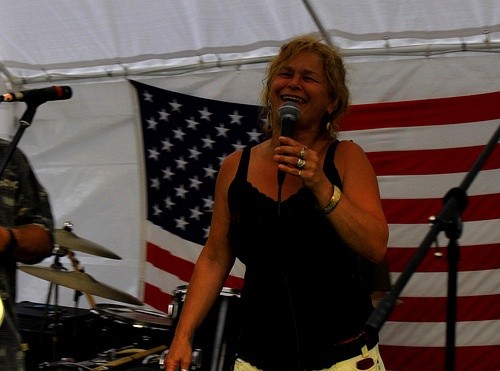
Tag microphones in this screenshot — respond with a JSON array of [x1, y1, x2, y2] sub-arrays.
[[278, 102, 301, 187], [0, 86, 72, 103]]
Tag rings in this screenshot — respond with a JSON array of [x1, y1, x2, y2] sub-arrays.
[[297, 143, 308, 176], [180, 368, 189, 371]]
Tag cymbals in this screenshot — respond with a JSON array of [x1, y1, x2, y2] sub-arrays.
[[48, 222, 123, 260], [16, 265, 145, 306]]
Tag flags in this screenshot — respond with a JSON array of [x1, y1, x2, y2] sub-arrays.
[[127, 75, 500, 371]]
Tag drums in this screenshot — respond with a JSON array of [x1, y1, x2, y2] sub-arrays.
[[170, 284, 245, 371], [90, 301, 175, 351], [36, 346, 169, 371]]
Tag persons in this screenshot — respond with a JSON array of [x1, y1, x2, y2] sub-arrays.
[[0, 137, 55, 370], [164, 34, 390, 370]]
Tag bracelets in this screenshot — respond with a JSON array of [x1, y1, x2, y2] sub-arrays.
[[4, 225, 19, 258], [321, 183, 341, 216]]
